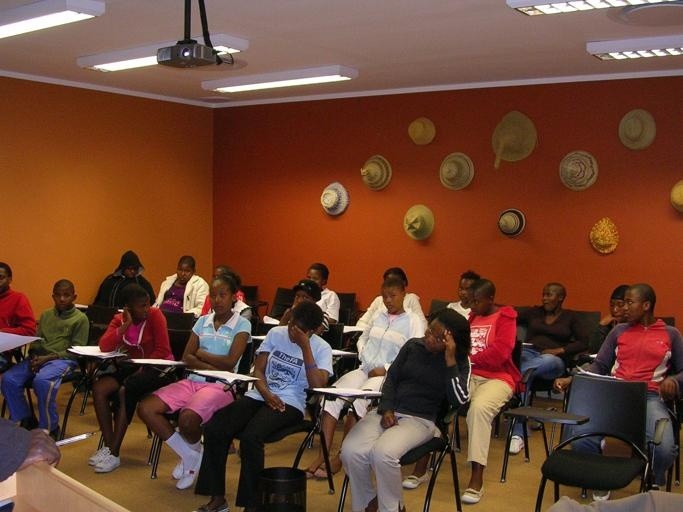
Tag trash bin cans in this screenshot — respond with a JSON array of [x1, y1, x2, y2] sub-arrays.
[[257, 468, 306, 512]]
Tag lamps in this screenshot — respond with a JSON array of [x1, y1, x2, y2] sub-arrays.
[[505, 0, 678, 16], [77, 34, 249, 72], [0, 0, 106, 39], [201, 65, 359, 93], [586, 35, 683, 61]]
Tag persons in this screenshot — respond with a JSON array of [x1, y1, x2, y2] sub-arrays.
[[357, 268, 428, 329], [278, 279, 330, 337], [88, 284, 174, 474], [207, 272, 252, 322], [552, 282, 683, 503], [135, 271, 253, 490], [1, 415, 60, 482], [338, 306, 472, 510], [400, 277, 522, 504], [152, 256, 209, 317], [306, 262, 340, 323], [447, 270, 481, 319], [94, 250, 156, 309], [302, 278, 425, 480], [0, 261, 37, 374], [400, 278, 522, 504], [591, 285, 629, 356], [202, 265, 247, 314], [191, 300, 333, 512], [1, 278, 90, 444], [510, 283, 589, 453]]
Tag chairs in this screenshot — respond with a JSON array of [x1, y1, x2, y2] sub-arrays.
[[34, 284, 683, 512]]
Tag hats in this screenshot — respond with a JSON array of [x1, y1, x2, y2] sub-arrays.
[[360, 155, 392, 191], [618, 108, 656, 151], [439, 152, 475, 191], [560, 150, 599, 192], [498, 209, 526, 237], [320, 181, 349, 215], [671, 180, 683, 212], [491, 110, 536, 170], [292, 279, 321, 300], [590, 216, 620, 255], [408, 117, 436, 146], [403, 205, 434, 240]]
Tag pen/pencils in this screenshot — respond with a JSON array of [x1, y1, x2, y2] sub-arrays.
[[56, 431, 96, 447], [118, 309, 124, 313]]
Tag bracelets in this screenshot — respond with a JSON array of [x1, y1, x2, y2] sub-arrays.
[[305, 364, 317, 369]]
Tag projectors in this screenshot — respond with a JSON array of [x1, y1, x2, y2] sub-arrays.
[[156, 38, 220, 69]]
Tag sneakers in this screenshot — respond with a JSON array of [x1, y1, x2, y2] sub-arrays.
[[88, 447, 111, 465], [20, 415, 38, 430], [402, 472, 429, 489], [172, 444, 204, 479], [461, 484, 485, 503], [49, 426, 60, 441], [530, 420, 543, 430], [508, 434, 525, 454], [592, 490, 611, 501], [192, 499, 230, 512], [305, 460, 325, 478], [176, 454, 202, 489], [314, 460, 342, 478], [95, 454, 121, 473]]
